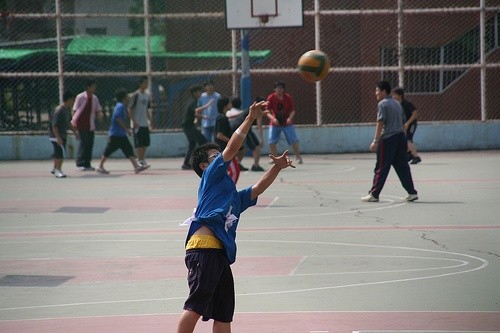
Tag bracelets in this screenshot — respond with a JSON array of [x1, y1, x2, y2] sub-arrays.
[[374, 140, 378, 143]]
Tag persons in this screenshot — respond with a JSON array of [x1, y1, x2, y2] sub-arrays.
[[264, 81, 303, 164], [96, 89, 146, 174], [361, 80, 419, 202], [215, 96, 240, 183], [182, 86, 208, 168], [71, 79, 102, 170], [127, 76, 153, 169], [391, 88, 421, 164], [233, 96, 264, 171], [197, 80, 221, 143], [226, 97, 243, 121], [176, 101, 296, 333], [48, 91, 75, 178]]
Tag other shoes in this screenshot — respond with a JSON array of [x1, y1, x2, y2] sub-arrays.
[[134, 164, 151, 174], [411, 156, 422, 164], [51, 168, 66, 178], [96, 168, 112, 175], [239, 164, 249, 171], [182, 164, 191, 169], [76, 164, 95, 171], [250, 165, 264, 171]]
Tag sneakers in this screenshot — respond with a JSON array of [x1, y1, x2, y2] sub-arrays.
[[361, 191, 379, 202], [406, 194, 418, 200]]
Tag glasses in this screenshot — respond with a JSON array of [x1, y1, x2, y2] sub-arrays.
[[208, 153, 220, 159]]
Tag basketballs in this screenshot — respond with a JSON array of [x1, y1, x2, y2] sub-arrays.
[[297, 49, 330, 83]]
